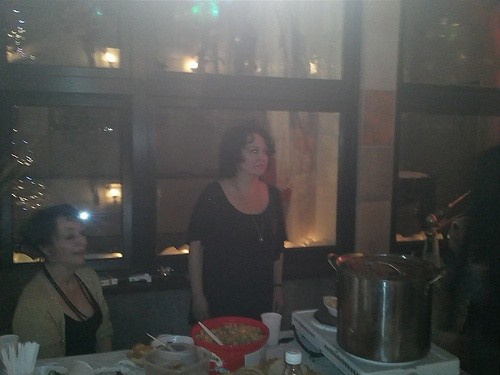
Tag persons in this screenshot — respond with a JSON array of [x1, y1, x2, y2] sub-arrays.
[[12, 204, 113, 359], [419, 214, 471, 297], [188, 124, 285, 325]]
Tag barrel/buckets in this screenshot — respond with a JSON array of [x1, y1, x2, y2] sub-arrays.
[[192, 317, 270, 375]]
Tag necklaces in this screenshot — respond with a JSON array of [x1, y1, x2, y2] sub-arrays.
[[246, 203, 266, 243], [42, 260, 102, 329]]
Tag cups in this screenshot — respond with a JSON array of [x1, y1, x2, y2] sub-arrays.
[[261, 313, 282, 347], [39, 364, 67, 375]]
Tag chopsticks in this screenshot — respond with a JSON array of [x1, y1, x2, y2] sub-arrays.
[[197, 321, 224, 348]]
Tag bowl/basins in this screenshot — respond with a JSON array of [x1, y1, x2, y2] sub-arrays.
[[152, 333, 195, 360], [322, 296, 338, 317], [0, 334, 21, 354]]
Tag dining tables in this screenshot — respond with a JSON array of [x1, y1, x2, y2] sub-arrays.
[[31, 330, 345, 375]]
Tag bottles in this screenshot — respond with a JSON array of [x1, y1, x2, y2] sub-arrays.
[[281, 349, 303, 375], [420, 189, 475, 231]]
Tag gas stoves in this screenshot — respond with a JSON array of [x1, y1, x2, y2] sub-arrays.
[[290, 308, 461, 375]]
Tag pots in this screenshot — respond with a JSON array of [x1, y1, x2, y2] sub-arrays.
[[326, 250, 448, 364]]
